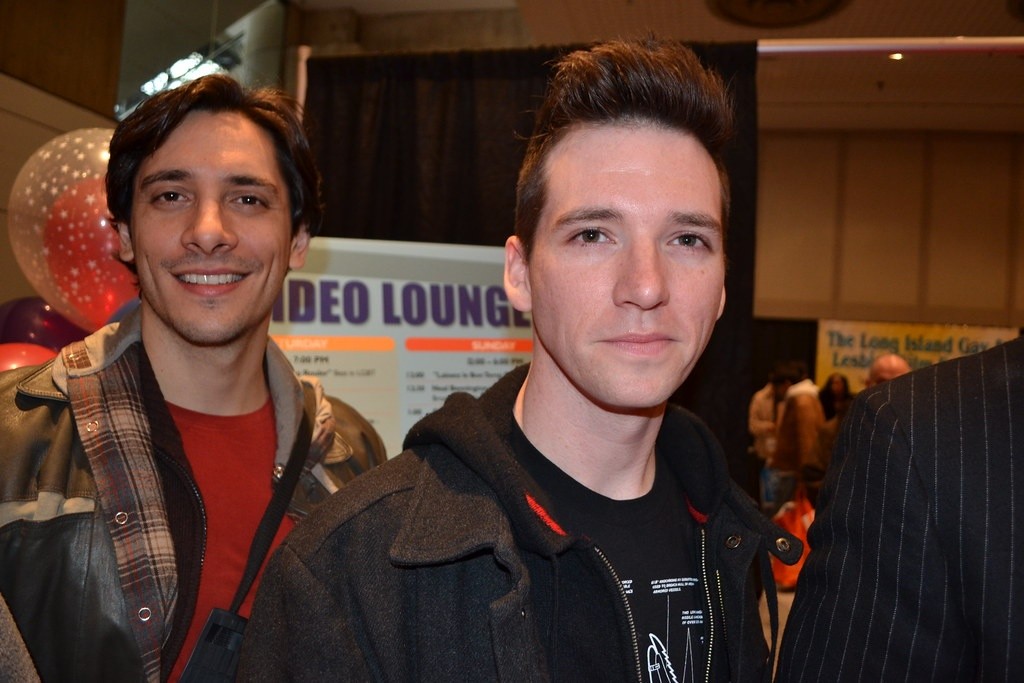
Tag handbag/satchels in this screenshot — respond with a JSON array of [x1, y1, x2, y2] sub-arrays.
[[767, 480, 816, 591]]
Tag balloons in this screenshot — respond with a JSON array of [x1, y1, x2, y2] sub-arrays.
[[0, 126, 142, 375]]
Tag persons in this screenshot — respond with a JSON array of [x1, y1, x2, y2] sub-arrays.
[[737, 347, 914, 509], [2, 75, 390, 683], [230, 37, 780, 683], [767, 334, 1024, 683]]
